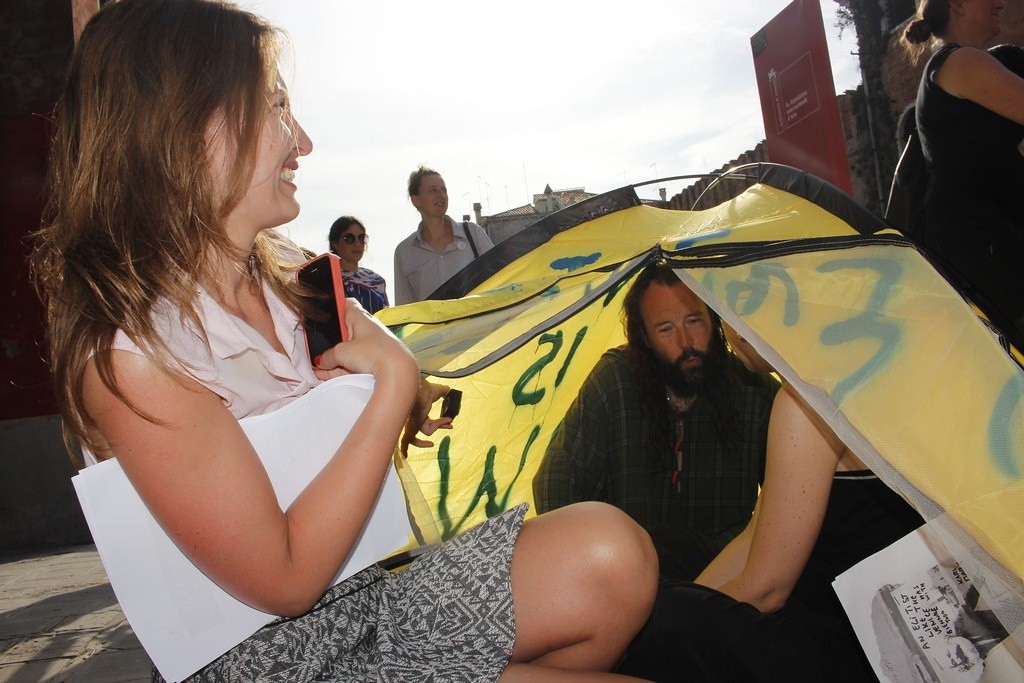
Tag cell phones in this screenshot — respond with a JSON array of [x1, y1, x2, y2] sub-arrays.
[[296, 253, 349, 371]]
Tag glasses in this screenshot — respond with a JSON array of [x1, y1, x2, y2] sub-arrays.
[[343, 232, 365, 244]]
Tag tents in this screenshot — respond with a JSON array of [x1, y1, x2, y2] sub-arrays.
[[374, 162, 1024, 683]]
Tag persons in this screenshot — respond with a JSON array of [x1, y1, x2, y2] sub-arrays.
[[532, 265, 782, 584], [394, 167, 494, 306], [692, 311, 925, 623], [329, 216, 389, 316], [27, 0, 659, 683], [885, 0, 1023, 354]]
